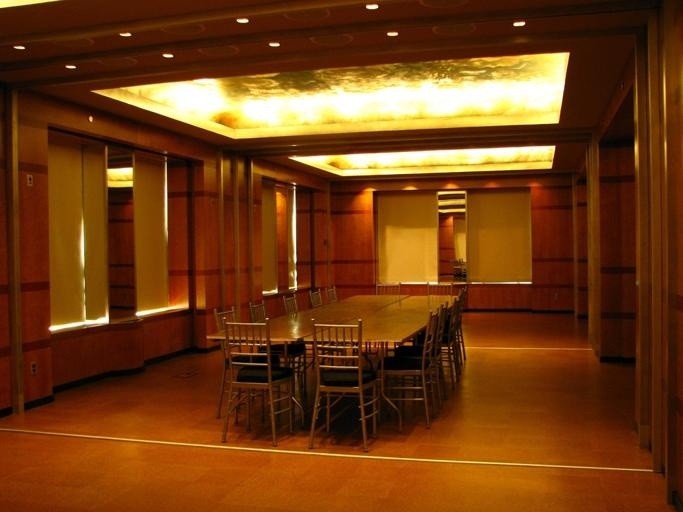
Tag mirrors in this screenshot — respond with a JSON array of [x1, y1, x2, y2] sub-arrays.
[[436, 190, 468, 282]]
